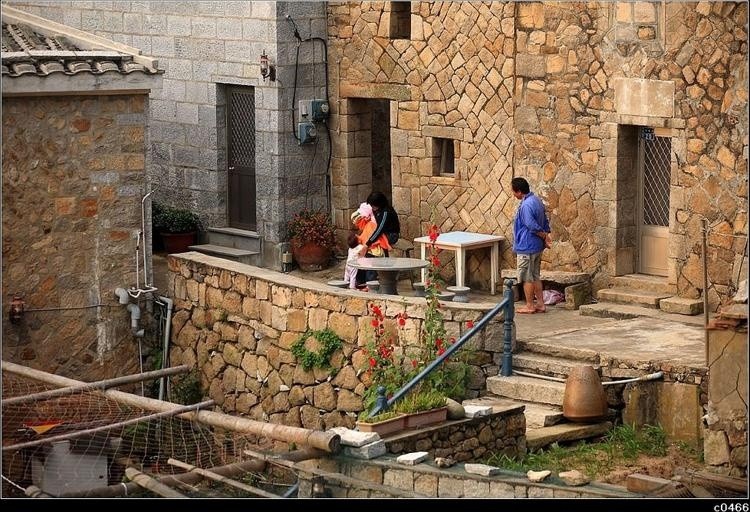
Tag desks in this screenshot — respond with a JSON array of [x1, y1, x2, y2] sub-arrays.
[[346, 230, 507, 296]]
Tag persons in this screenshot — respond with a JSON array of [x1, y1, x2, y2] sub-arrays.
[[343, 234, 363, 290], [511, 177, 555, 314], [357, 188, 402, 293]]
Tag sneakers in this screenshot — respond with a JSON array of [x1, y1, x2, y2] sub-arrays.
[[362, 287, 368, 291]]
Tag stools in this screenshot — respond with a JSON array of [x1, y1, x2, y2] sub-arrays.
[[327, 279, 473, 304]]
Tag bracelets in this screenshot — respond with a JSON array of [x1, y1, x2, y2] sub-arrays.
[[543, 235, 547, 242]]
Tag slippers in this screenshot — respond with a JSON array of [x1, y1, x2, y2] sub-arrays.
[[536, 310, 545, 313], [516, 308, 536, 314]]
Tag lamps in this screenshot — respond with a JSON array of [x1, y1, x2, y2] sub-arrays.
[[259, 47, 276, 82]]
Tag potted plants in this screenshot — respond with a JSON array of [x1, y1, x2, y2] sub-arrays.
[[285, 203, 346, 272], [355, 389, 452, 436], [153, 207, 204, 254]]
